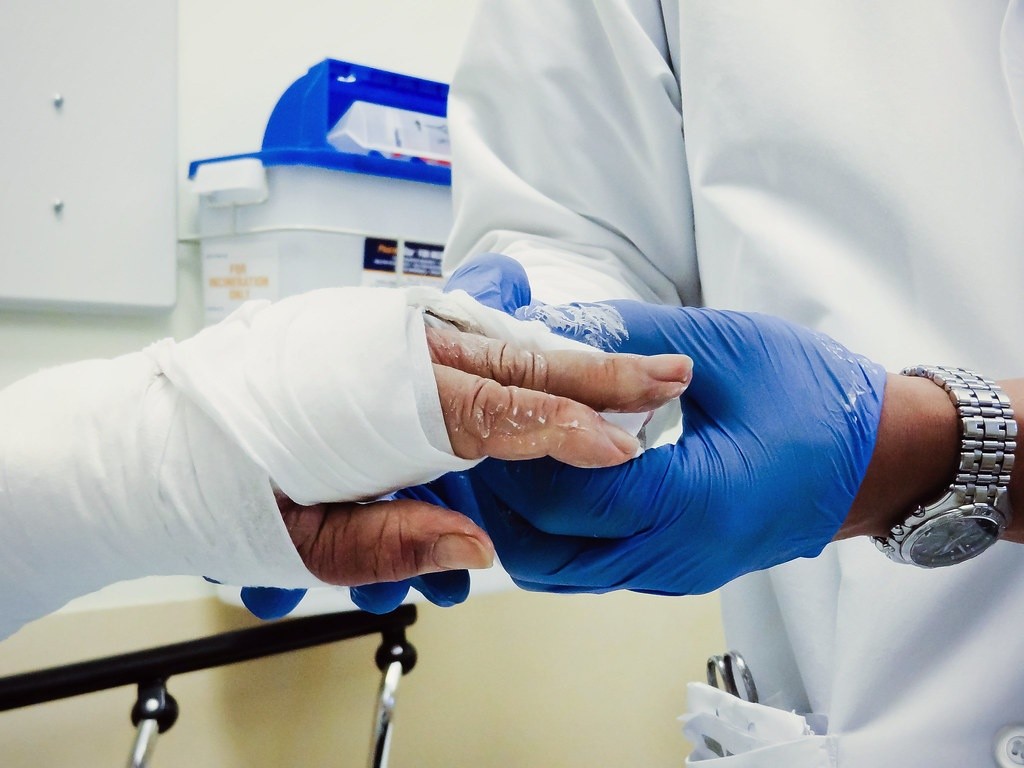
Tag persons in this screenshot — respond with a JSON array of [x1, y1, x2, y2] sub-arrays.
[[0, 287, 693, 654], [203, 0, 1024, 768]]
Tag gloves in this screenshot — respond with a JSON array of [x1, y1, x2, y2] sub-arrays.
[[467, 297, 888, 596], [203, 254, 533, 619]]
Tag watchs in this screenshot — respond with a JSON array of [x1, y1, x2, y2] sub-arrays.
[[867, 362, 1014, 571]]
